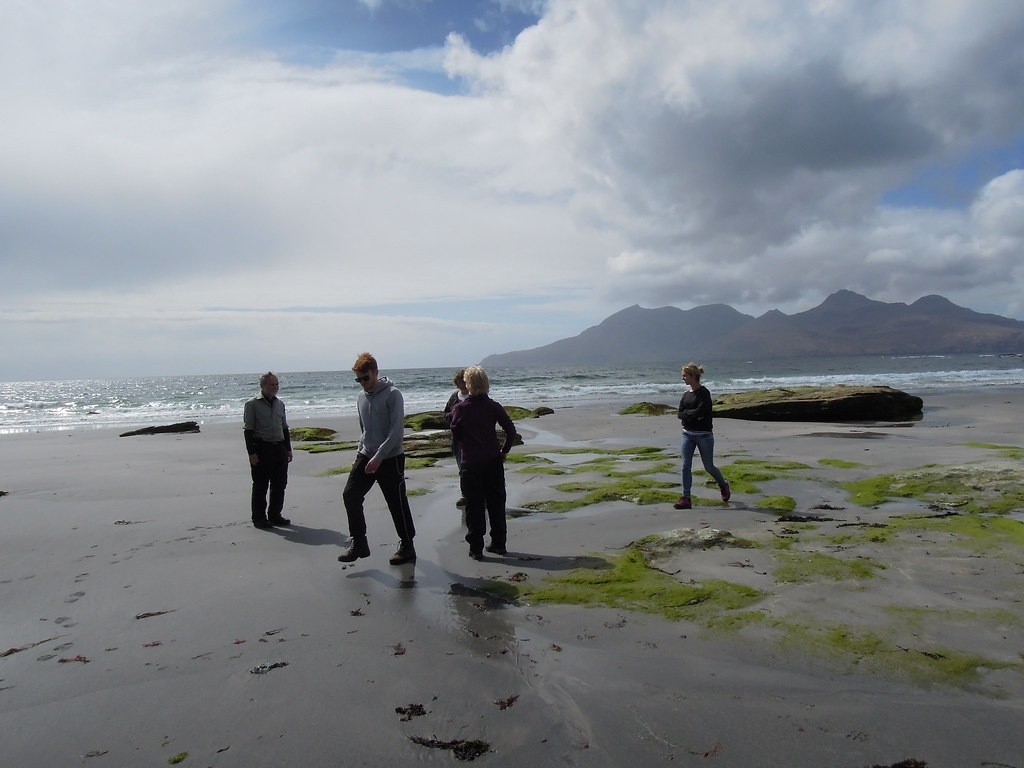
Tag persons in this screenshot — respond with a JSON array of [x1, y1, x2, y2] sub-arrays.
[[444, 367, 517, 559], [339, 353, 416, 565], [243, 372, 293, 530], [673, 362, 731, 509]]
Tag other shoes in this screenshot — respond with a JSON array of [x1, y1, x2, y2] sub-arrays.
[[268, 513, 290, 526], [486, 546, 506, 555], [469, 551, 482, 560], [252, 514, 273, 528], [456, 498, 466, 506]]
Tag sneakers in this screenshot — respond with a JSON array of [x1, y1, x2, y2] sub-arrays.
[[389, 540, 416, 565], [338, 536, 370, 562], [674, 496, 691, 509], [718, 482, 730, 502]]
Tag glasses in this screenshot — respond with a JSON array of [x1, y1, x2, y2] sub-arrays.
[[355, 375, 369, 382]]
